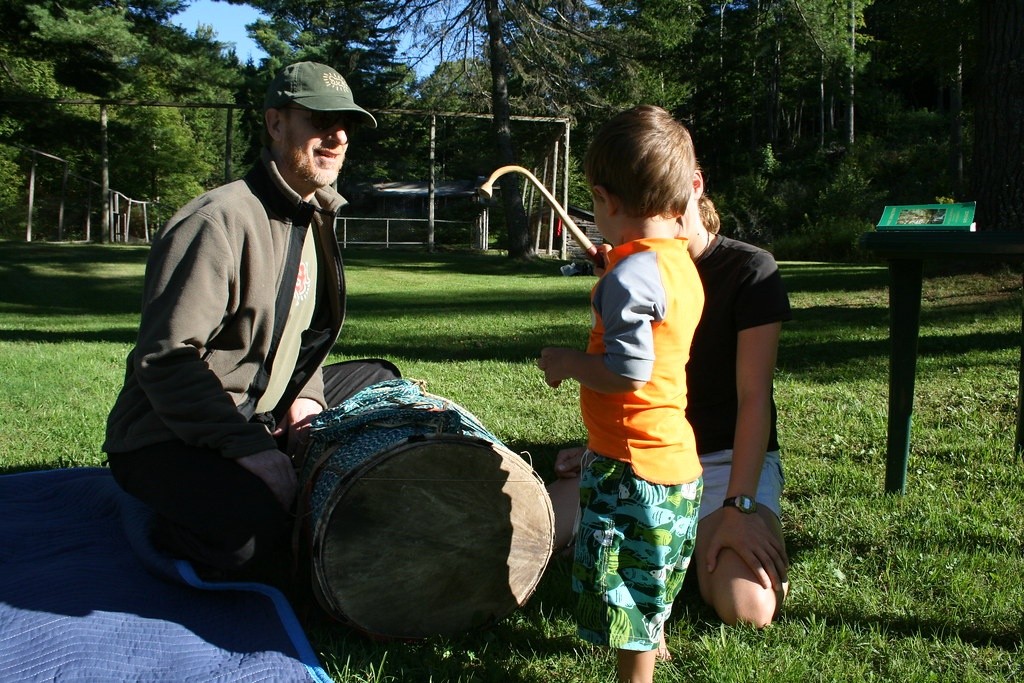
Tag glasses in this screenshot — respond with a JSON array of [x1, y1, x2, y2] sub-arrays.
[[287, 107, 360, 132]]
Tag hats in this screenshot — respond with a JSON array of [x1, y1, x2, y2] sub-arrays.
[[263, 60, 377, 129]]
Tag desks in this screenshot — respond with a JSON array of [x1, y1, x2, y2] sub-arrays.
[[858, 232, 1024, 497]]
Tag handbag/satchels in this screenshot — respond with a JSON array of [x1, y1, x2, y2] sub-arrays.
[[299, 378, 555, 639]]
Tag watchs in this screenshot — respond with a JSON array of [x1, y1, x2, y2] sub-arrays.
[[721, 495, 755, 515]]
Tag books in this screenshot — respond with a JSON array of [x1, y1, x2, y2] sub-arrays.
[[876, 200, 978, 232]]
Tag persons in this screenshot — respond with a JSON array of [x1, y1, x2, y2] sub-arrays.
[[546, 157, 790, 630], [538, 106, 707, 683], [101, 59, 402, 667]]
